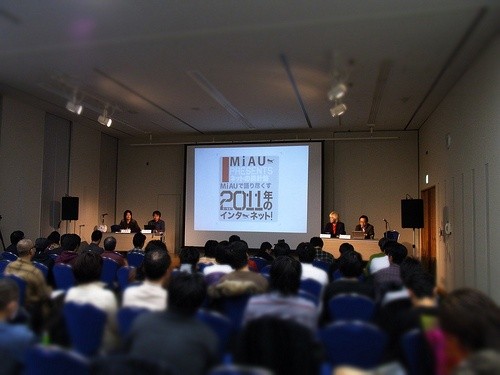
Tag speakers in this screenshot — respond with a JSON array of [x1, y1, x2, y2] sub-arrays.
[[400, 199, 424, 228], [61, 197, 79, 220]]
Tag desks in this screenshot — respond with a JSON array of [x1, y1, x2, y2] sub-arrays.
[[98, 232, 165, 251], [321, 237, 383, 261]]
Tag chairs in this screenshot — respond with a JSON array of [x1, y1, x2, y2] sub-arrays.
[[0, 248, 438, 375]]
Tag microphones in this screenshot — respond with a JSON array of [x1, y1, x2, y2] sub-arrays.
[[383, 218, 388, 223]]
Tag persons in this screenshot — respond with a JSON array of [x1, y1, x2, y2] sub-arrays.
[[120, 210, 141, 232], [325, 211, 347, 236], [0, 231, 499, 375], [147, 210, 166, 233], [354, 216, 373, 240]]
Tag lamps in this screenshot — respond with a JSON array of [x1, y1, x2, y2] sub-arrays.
[[330, 100, 347, 118], [97, 104, 113, 127], [326, 79, 348, 102], [66, 93, 83, 115]]
